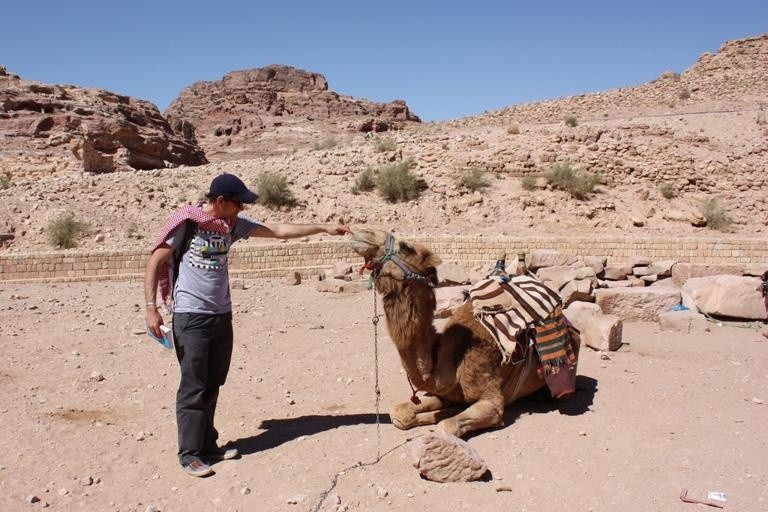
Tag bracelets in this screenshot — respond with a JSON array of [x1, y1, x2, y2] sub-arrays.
[[145, 302, 156, 305]]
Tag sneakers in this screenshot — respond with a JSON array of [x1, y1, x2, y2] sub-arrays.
[[182, 448, 238, 477]]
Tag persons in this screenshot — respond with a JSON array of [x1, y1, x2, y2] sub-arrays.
[[145, 172, 356, 477]]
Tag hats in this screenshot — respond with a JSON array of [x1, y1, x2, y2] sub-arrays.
[[210, 174, 259, 203]]
